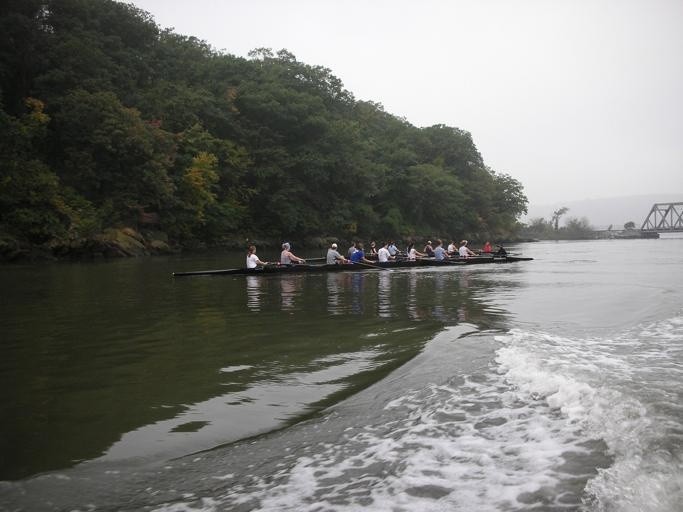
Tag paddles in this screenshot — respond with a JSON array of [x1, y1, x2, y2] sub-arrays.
[[347, 260, 388, 270]]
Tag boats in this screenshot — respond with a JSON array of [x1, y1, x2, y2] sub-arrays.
[[169, 248, 535, 278]]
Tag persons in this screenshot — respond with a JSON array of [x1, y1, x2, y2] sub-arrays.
[[326, 243, 350, 265], [245, 246, 268, 269], [347, 239, 402, 264], [447, 240, 457, 255], [279, 242, 305, 265], [406, 242, 424, 261], [458, 240, 479, 257], [491, 245, 507, 258], [423, 240, 434, 257], [434, 239, 449, 260]]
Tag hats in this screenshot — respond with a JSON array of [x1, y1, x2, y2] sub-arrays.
[[358, 243, 363, 249], [332, 243, 337, 247], [428, 240, 432, 244], [371, 241, 375, 247]]
